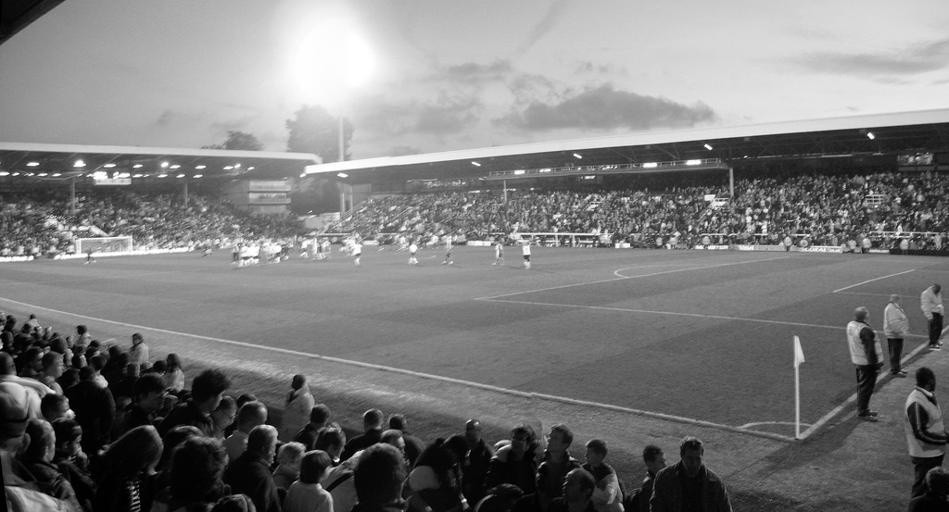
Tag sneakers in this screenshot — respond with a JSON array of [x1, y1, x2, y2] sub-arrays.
[[858, 410, 879, 422], [928, 340, 944, 351], [888, 369, 908, 378]]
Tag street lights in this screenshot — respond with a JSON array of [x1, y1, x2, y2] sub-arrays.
[[311, 36, 359, 213]]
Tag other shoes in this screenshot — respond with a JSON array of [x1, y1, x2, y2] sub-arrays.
[[491, 262, 496, 265], [233, 260, 361, 268], [407, 257, 418, 264], [525, 262, 530, 269], [442, 260, 454, 265]]
